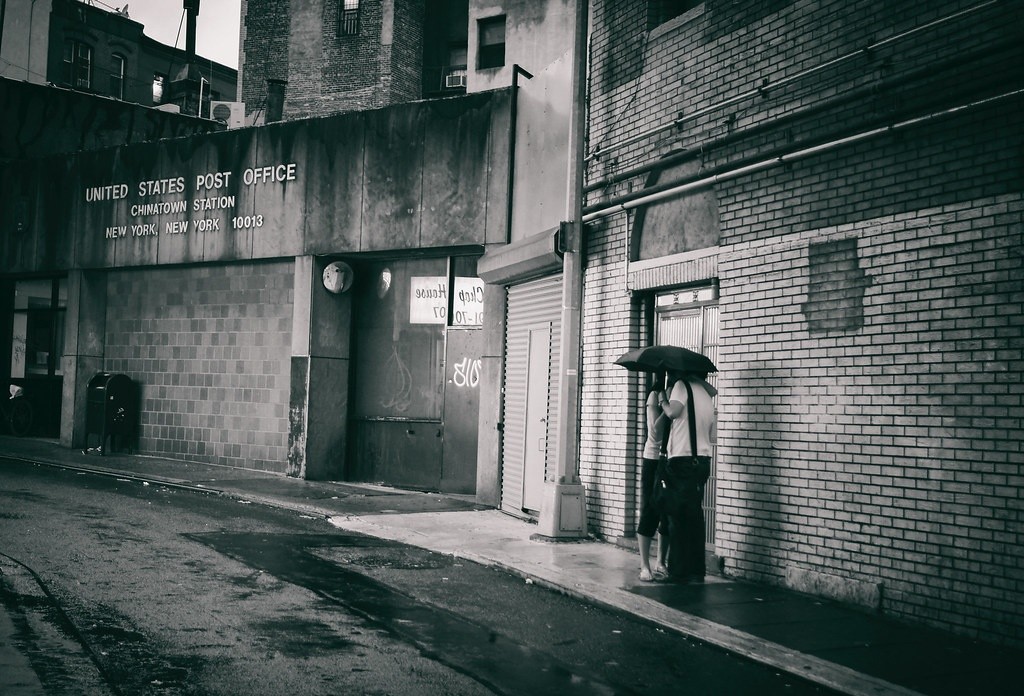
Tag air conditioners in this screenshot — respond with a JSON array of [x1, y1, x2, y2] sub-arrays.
[[446, 74, 467, 88], [210, 101, 245, 130]]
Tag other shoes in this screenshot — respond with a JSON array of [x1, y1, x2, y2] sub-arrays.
[[652, 566, 669, 577], [640, 571, 653, 581], [690, 575, 704, 583], [654, 573, 690, 583]]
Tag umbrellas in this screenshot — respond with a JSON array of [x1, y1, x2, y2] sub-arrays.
[[614, 345, 718, 373]]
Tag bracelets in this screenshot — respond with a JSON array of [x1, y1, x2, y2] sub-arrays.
[[658, 398, 666, 406]]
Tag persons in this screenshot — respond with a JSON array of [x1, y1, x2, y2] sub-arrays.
[[636, 372, 718, 586]]
